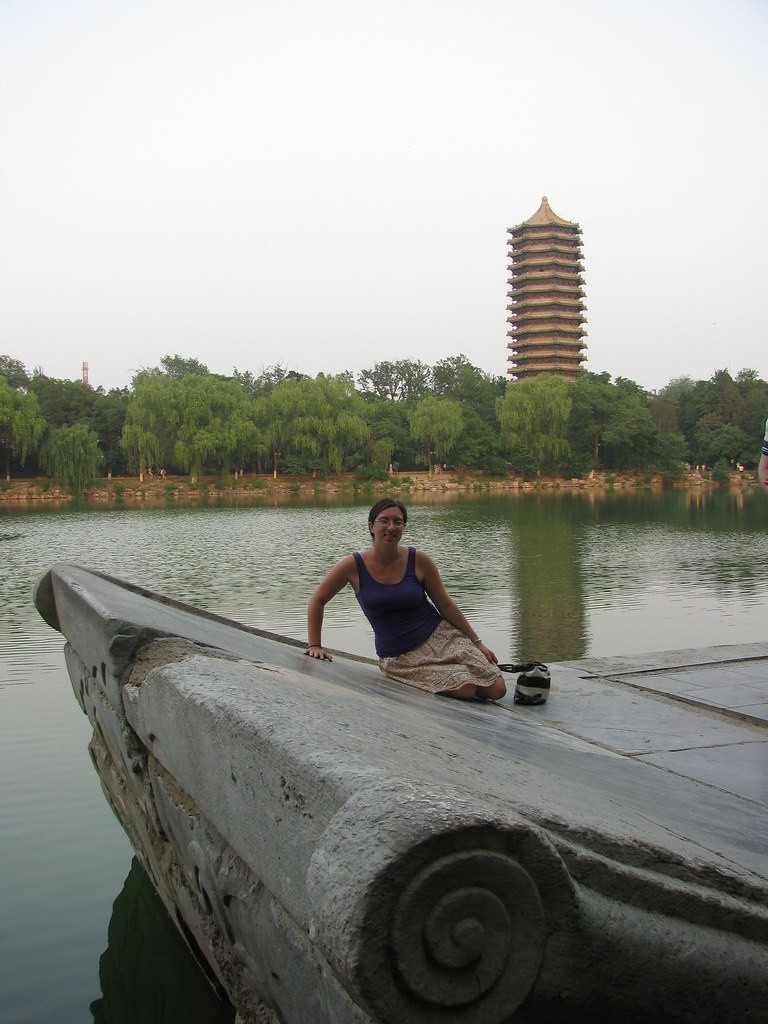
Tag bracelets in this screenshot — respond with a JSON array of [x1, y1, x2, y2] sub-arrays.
[[473, 639, 482, 642], [308, 645, 322, 649]]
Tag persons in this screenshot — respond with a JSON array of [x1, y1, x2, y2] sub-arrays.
[[758, 419, 768, 494], [302, 498, 507, 701]]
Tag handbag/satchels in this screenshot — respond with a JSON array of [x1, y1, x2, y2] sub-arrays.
[[496, 659, 551, 706]]
